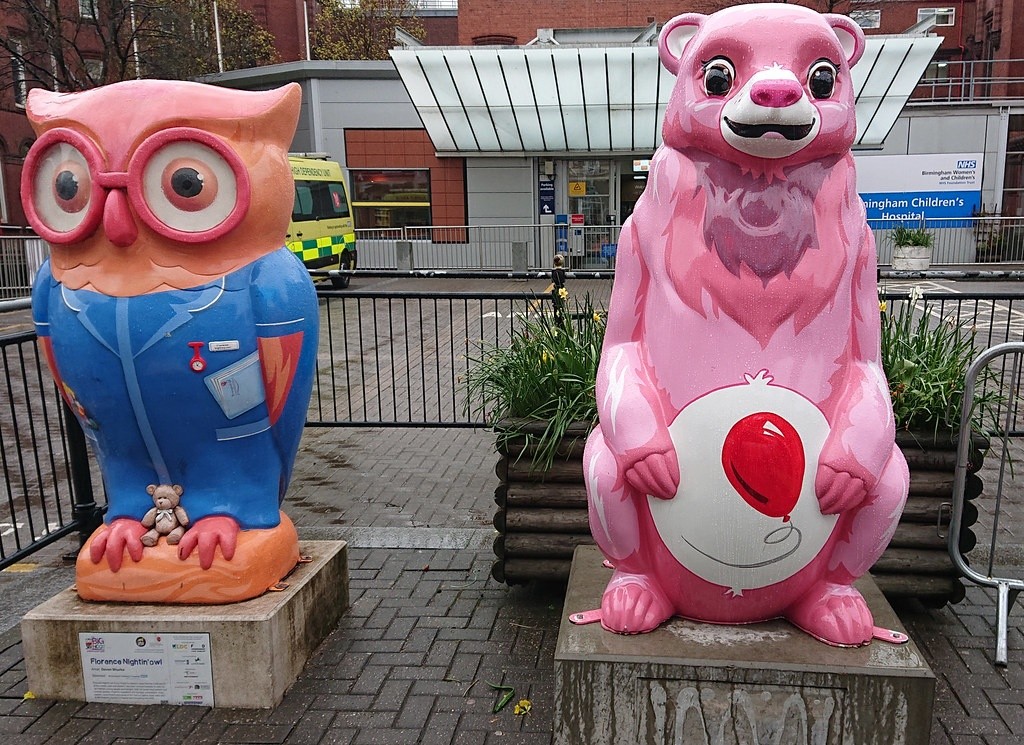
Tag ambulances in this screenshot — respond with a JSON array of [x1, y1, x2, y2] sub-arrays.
[[284, 151, 358, 289]]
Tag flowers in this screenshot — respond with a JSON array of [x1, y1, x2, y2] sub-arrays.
[[456, 275, 1018, 479]]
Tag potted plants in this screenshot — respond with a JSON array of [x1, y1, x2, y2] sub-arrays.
[[881, 226, 938, 271]]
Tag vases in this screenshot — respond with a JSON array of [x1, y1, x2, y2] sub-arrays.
[[488, 406, 990, 616]]
